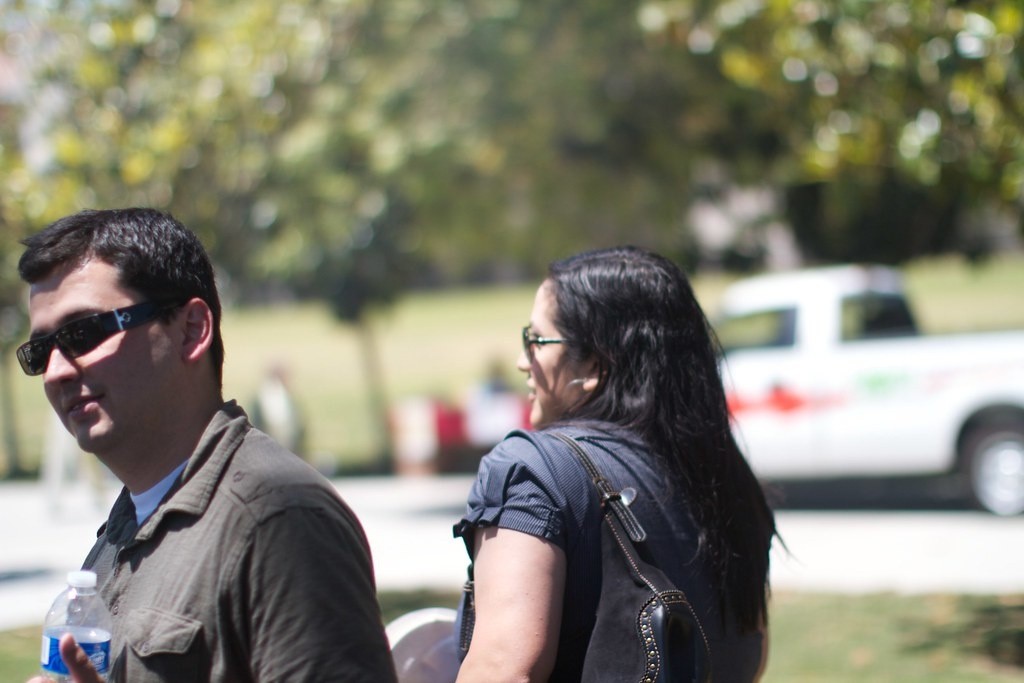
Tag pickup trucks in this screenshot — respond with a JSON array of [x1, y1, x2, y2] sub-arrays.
[[715, 264, 1024, 517]]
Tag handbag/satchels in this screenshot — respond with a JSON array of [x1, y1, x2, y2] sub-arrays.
[[459, 433, 711, 683]]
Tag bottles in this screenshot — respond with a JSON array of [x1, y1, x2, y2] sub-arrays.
[[37, 571, 115, 682]]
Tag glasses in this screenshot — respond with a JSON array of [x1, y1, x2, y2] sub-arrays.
[[522, 326, 571, 364], [16, 296, 186, 376]]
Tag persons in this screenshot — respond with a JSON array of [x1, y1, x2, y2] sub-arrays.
[[15, 208, 399, 683], [455, 245, 774, 683]]
